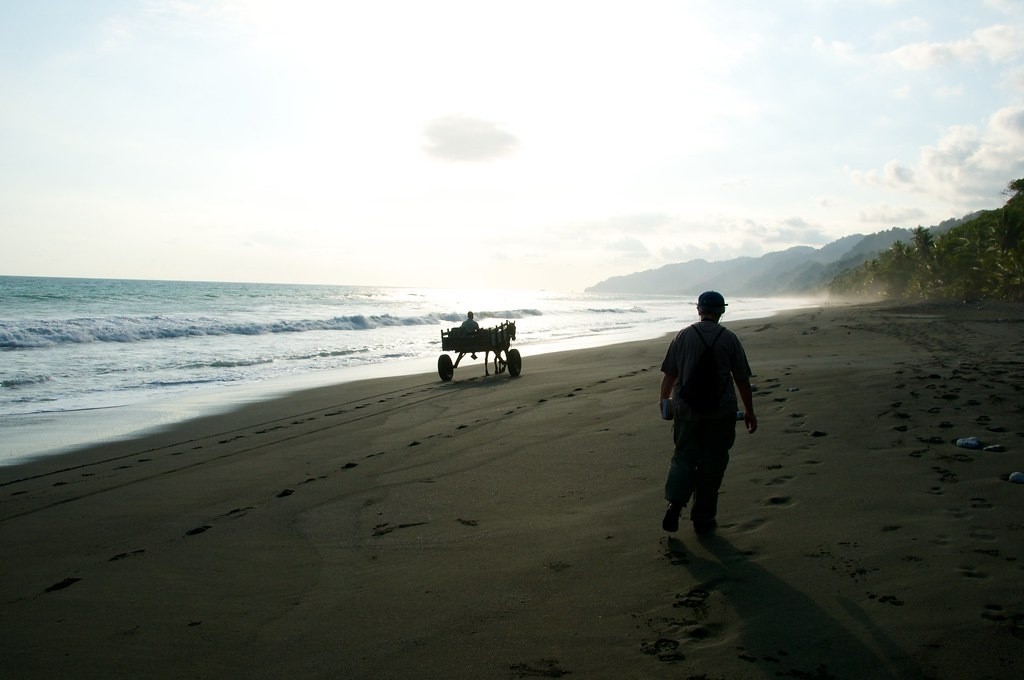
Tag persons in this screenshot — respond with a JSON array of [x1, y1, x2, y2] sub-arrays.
[[659, 290, 757, 537]]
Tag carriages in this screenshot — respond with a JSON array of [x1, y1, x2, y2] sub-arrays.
[[438, 321, 522, 380]]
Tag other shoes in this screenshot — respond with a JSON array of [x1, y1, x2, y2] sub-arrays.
[[662, 503, 679, 532], [693, 520, 717, 538]]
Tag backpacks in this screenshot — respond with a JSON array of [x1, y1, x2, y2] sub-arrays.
[[683, 323, 730, 415]]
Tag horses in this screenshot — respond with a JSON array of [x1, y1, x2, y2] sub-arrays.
[[495, 320, 516, 375]]
